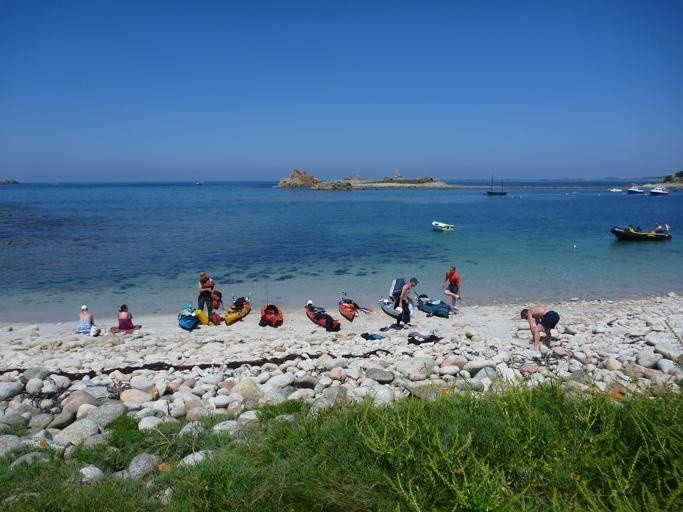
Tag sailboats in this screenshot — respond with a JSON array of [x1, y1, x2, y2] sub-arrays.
[[487, 172, 507, 195]]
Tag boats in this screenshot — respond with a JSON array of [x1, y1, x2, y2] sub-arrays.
[[261, 304, 283, 326], [380, 296, 403, 317], [339, 296, 356, 320], [608, 184, 669, 195], [611, 224, 671, 241], [432, 220, 455, 232], [225, 297, 251, 325], [417, 294, 449, 318], [305, 300, 338, 330]]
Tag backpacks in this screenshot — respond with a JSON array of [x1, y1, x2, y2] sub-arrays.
[[210, 291, 221, 309]]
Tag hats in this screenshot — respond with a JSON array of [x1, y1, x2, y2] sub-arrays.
[[410, 278, 418, 283], [199, 272, 206, 280], [81, 305, 87, 311]]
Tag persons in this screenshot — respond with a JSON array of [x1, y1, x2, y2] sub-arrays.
[[337, 291, 361, 310], [623, 225, 637, 233], [116, 303, 133, 329], [305, 298, 333, 331], [391, 277, 420, 330], [78, 305, 94, 335], [441, 265, 461, 311], [519, 306, 560, 351], [195, 271, 215, 326], [650, 222, 662, 233], [230, 295, 247, 311]]
